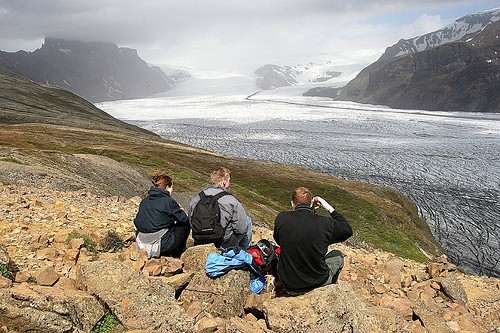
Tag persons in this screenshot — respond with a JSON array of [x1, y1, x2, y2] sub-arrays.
[[188, 166, 253, 250], [274, 187, 353, 296], [133, 174, 191, 259]]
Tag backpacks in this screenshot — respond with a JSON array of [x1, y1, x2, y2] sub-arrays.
[[190, 190, 233, 244], [239, 238, 280, 279]]
